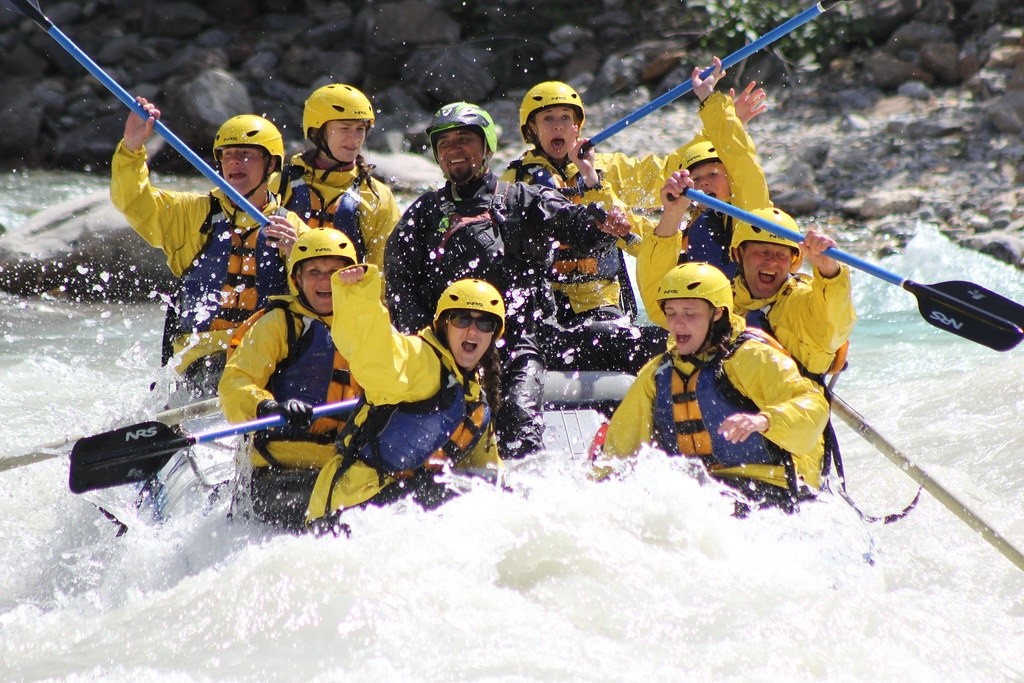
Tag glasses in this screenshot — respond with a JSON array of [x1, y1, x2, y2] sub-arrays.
[[445, 309, 498, 332]]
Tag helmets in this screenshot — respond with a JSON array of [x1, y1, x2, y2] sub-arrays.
[[425, 101, 497, 165], [728, 207, 801, 267], [678, 141, 723, 173], [302, 82, 375, 140], [287, 226, 358, 297], [433, 279, 505, 341], [655, 262, 734, 326], [519, 81, 586, 144], [213, 113, 284, 172]]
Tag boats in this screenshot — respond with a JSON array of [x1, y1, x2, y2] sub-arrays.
[[123, 370, 640, 533]]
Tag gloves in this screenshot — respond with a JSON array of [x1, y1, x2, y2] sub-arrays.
[[263, 399, 314, 427]]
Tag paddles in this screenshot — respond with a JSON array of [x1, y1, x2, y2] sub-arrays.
[[69, 398, 361, 495], [666, 186, 1023, 354], [557, 0, 840, 173], [746, 310, 1024, 573], [0, 0, 271, 230]]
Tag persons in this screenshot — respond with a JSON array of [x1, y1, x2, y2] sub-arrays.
[[382, 102, 631, 461], [637, 167, 853, 390], [569, 56, 774, 284], [109, 94, 314, 415], [304, 262, 510, 541], [277, 82, 404, 312], [217, 225, 367, 540], [582, 260, 831, 521], [500, 81, 767, 331]]
